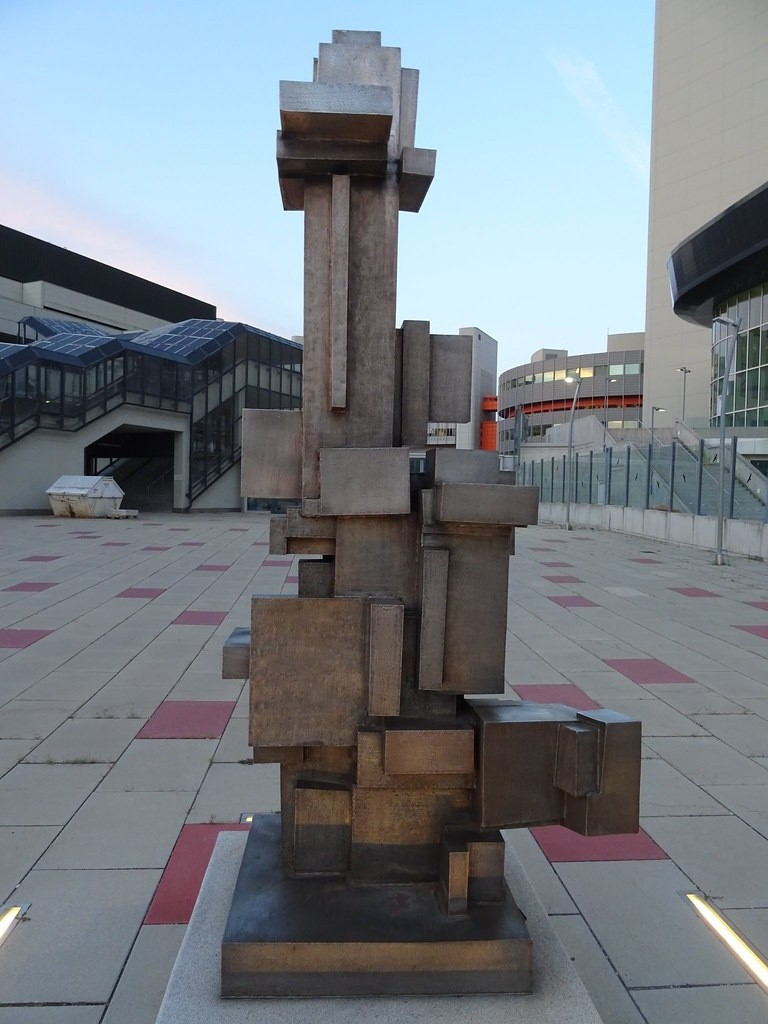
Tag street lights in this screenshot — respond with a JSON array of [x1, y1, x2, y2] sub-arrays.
[[603, 375, 618, 449], [564, 377, 584, 530], [675, 366, 692, 421], [650, 405, 667, 445], [711, 316, 744, 565]]
